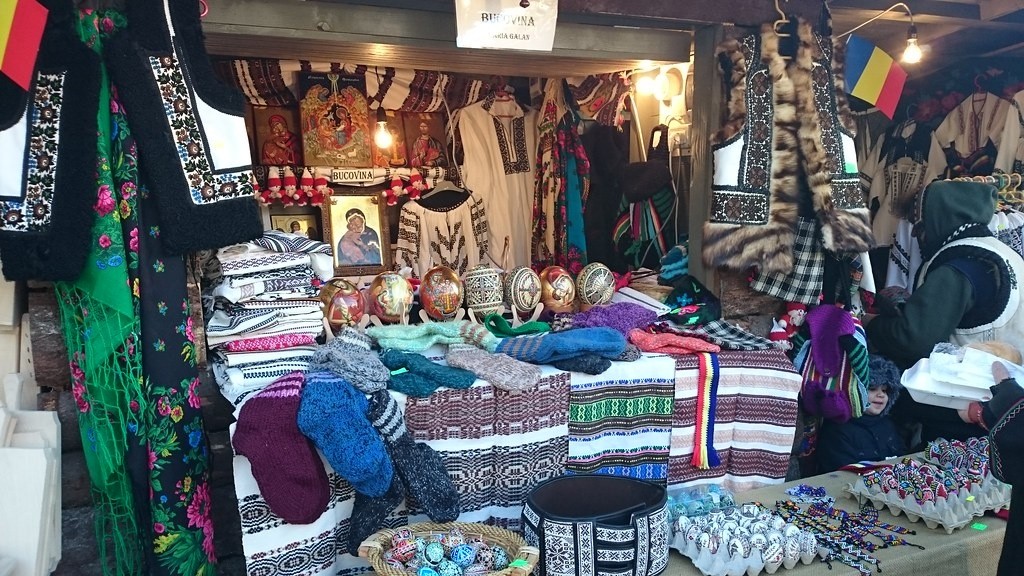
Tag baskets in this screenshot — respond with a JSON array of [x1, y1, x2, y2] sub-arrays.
[[359, 521, 539, 575]]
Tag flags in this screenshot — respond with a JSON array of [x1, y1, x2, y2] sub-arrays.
[[841, 34, 909, 119]]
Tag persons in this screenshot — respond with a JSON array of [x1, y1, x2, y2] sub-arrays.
[[955, 360, 1024, 576], [817, 353, 910, 474], [860, 178, 1023, 441]]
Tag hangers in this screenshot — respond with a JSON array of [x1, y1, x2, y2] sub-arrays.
[[665, 115, 684, 128], [483, 74, 517, 118], [973, 72, 989, 102], [717, 0, 796, 75], [890, 144, 918, 174], [200, 0, 209, 19], [538, 90, 558, 128], [943, 172, 1024, 209], [421, 169, 465, 200], [902, 103, 918, 139]]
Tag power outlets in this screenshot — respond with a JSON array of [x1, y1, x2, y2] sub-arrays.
[[669, 123, 693, 149]]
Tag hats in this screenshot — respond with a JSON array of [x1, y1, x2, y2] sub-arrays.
[[867, 353, 900, 416]]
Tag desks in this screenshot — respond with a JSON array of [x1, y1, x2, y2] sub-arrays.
[[656, 451, 1008, 576]]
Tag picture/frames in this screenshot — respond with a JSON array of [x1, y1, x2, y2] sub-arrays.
[[252, 104, 303, 166], [321, 186, 393, 278], [294, 70, 374, 169], [268, 204, 324, 243], [368, 109, 409, 169], [402, 111, 450, 167]]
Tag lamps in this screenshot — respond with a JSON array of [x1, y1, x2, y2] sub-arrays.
[[838, 3, 923, 64], [635, 68, 682, 106]]
